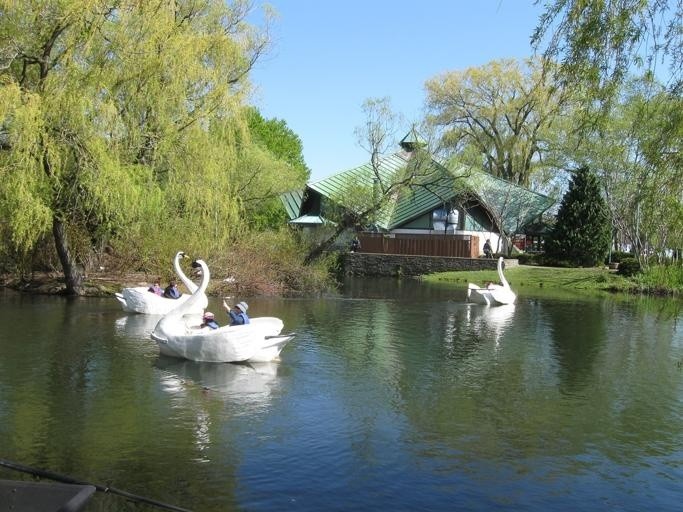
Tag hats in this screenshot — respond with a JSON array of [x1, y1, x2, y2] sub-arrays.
[[234, 302, 248, 313], [170, 281, 176, 286], [202, 312, 214, 319]]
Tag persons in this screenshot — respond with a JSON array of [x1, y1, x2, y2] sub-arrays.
[[349, 236, 360, 254], [147, 280, 164, 298], [484, 280, 492, 291], [163, 281, 182, 299], [199, 311, 219, 330], [221, 298, 249, 327], [483, 239, 494, 259]]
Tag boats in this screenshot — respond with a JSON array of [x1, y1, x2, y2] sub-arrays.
[[114, 251, 208, 315], [150, 259, 296, 364], [466, 256, 515, 308]]
